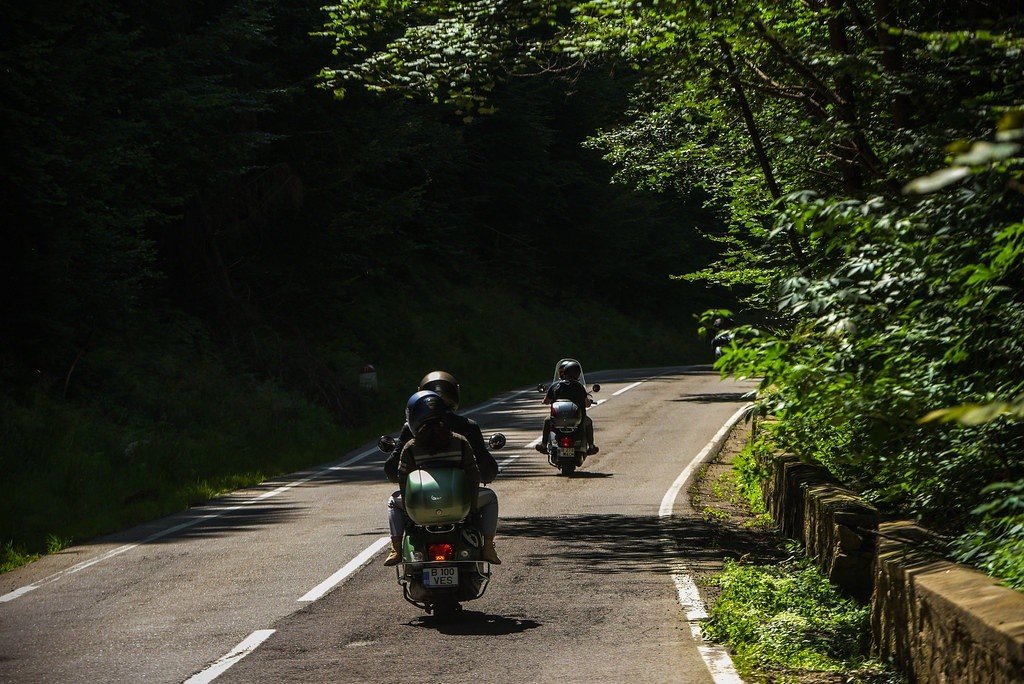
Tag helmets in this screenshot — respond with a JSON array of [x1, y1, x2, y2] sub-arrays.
[[564, 361, 581, 379], [405, 389, 452, 438], [417, 370, 461, 412], [559, 360, 569, 375]]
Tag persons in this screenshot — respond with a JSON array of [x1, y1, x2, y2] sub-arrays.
[[535, 361, 599, 456], [384, 371, 501, 566]]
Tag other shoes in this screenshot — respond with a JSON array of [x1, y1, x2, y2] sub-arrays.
[[535, 444, 549, 454], [586, 445, 599, 455]]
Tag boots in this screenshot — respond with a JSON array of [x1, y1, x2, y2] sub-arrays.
[[383, 540, 403, 566], [481, 538, 501, 564]]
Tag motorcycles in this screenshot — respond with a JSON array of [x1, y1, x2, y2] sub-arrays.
[[538, 384, 601, 476], [376, 433, 507, 622]]
